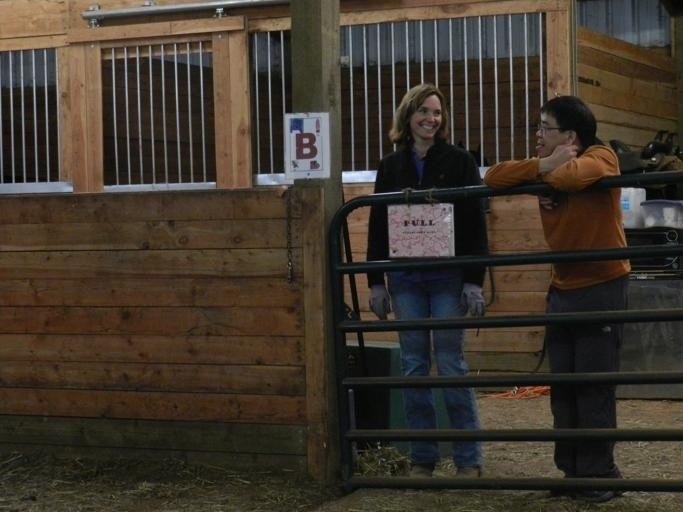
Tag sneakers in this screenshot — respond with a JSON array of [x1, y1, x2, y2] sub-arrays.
[[454, 466, 481, 479], [548, 463, 624, 502], [410, 463, 435, 477]]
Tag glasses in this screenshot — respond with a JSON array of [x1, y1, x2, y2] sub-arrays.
[[535, 122, 563, 136]]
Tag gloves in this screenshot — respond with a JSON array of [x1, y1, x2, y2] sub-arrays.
[[368, 283, 391, 320], [461, 281, 487, 316]]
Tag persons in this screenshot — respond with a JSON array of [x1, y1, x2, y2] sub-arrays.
[[484, 95, 628, 504], [366, 83, 488, 478]]
